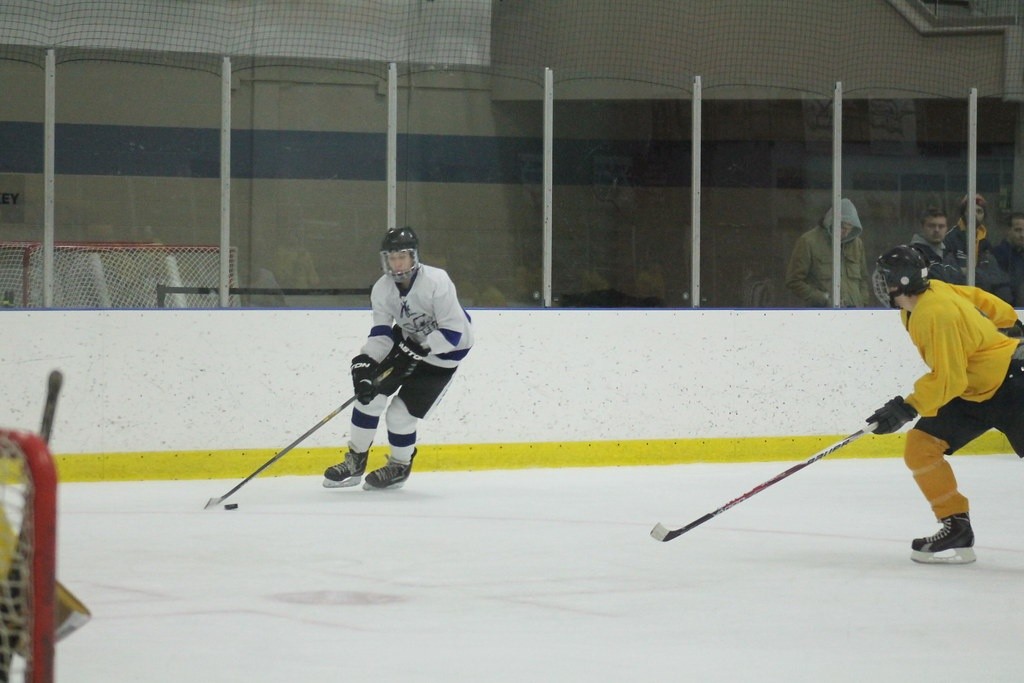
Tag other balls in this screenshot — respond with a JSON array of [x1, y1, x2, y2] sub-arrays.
[[224, 503, 238, 511]]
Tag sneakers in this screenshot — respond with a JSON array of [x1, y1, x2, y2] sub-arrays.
[[910, 512, 977, 564], [323, 440, 373, 488], [362, 447, 418, 491]]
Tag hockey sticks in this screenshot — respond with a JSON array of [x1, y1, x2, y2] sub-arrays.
[[205, 391, 359, 508], [0, 370, 63, 683], [650, 423, 877, 542]]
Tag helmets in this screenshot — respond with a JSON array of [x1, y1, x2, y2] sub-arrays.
[[379, 226, 419, 282], [872, 245, 929, 310]]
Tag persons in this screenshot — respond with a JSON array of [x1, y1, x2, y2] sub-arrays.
[[322, 225, 474, 488], [783, 197, 869, 309], [907, 194, 1024, 309], [866, 243, 1024, 554]]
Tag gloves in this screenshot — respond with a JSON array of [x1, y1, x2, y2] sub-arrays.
[[866, 395, 918, 435], [350, 354, 380, 406], [998, 319, 1024, 338], [389, 336, 431, 381]]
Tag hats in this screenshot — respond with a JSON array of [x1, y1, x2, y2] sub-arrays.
[[961, 192, 987, 208]]
